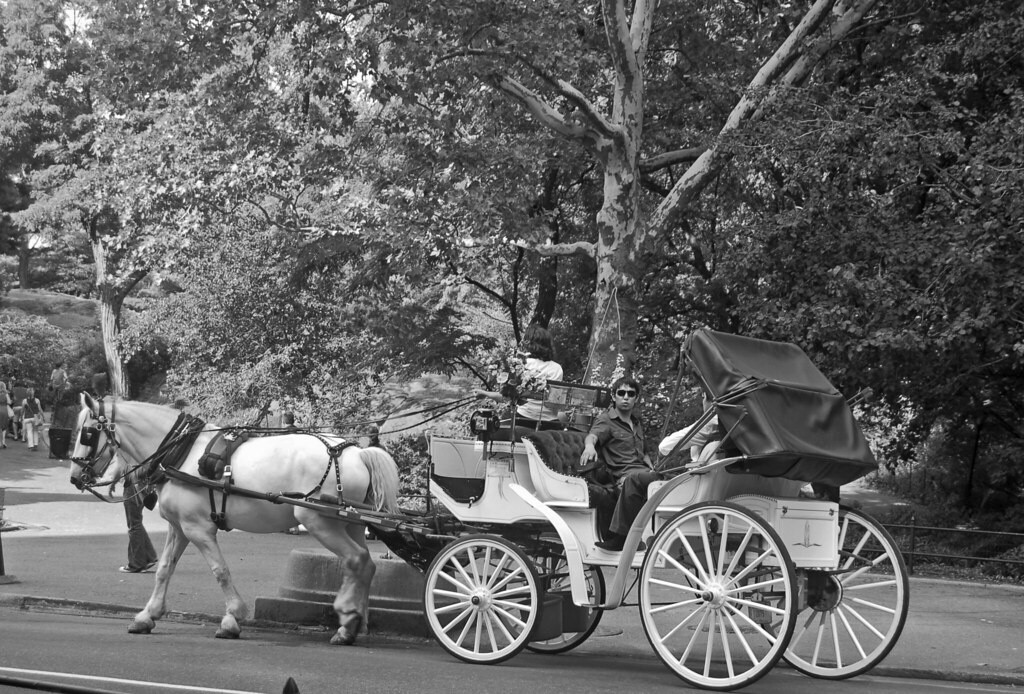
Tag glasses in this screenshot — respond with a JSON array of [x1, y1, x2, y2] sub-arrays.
[[616, 389, 637, 397]]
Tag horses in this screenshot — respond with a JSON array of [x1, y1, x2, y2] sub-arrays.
[[69, 391, 402, 645]]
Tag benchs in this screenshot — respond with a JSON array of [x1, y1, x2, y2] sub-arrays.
[[519, 430, 601, 510], [474, 414, 588, 456], [640, 443, 808, 545]]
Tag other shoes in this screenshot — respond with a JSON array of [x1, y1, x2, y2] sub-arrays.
[[0, 575, 17, 584], [594, 534, 646, 550], [119, 560, 156, 573]]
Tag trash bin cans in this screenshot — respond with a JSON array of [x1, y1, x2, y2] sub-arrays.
[[48, 427, 73, 459]]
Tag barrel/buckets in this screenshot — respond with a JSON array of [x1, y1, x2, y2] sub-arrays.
[[554, 572, 593, 632], [520, 592, 562, 642]]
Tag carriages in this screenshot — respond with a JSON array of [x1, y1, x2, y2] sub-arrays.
[[69, 328, 908, 691]]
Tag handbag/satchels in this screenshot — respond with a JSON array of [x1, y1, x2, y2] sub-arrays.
[[6, 404, 15, 419], [33, 413, 44, 426]]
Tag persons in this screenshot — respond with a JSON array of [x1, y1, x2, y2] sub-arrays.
[[579, 376, 719, 551], [474, 324, 564, 421], [48, 362, 82, 459], [283, 411, 295, 428], [0, 373, 44, 449]]
